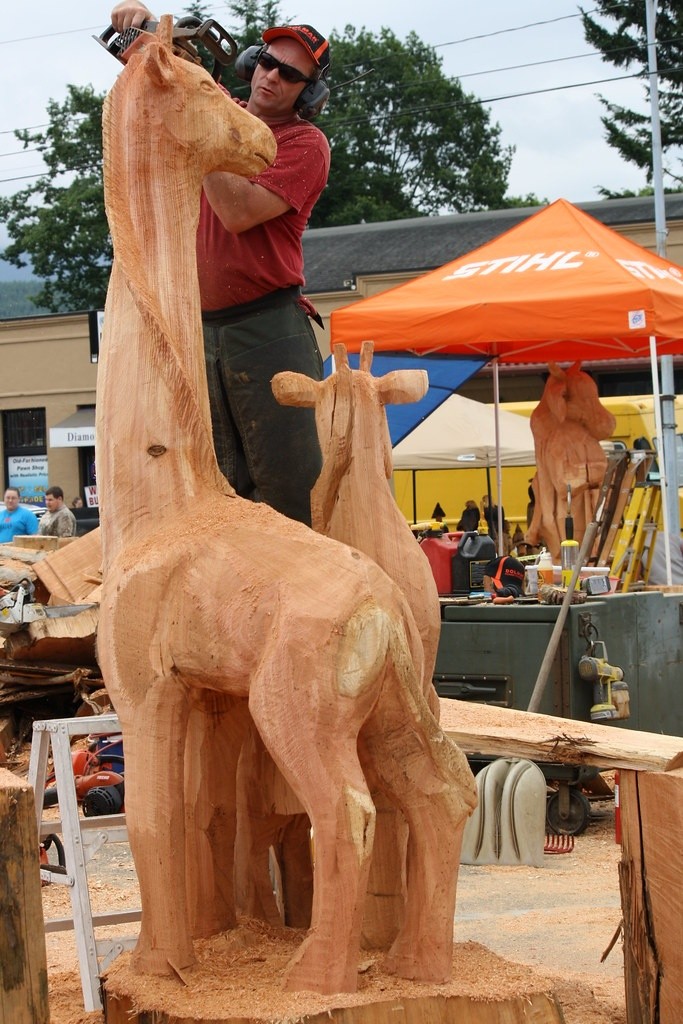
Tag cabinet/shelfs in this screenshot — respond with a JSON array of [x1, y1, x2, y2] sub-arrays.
[[432, 589, 683, 836]]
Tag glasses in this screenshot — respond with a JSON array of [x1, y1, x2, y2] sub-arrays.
[[257, 51, 313, 84]]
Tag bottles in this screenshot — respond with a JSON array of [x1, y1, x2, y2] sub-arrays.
[[560, 517, 580, 591], [536, 553, 554, 604]]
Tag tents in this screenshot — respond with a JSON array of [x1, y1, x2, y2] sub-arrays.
[[329, 197, 682, 592]]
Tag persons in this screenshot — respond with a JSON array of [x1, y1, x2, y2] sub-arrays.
[[526, 485, 535, 529], [456, 500, 481, 531], [482, 495, 505, 541], [111, 0, 332, 528], [72, 497, 83, 508], [0, 486, 40, 544], [38, 486, 77, 537]]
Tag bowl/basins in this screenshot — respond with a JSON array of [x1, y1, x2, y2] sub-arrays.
[[525, 564, 610, 593]]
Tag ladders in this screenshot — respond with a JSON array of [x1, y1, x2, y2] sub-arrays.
[[27, 713, 140, 1011]]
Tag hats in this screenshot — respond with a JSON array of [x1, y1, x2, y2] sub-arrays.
[[261, 24, 332, 80], [484, 554, 524, 593]]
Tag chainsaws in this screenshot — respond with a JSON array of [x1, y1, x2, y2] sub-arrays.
[[91, 0, 238, 89]]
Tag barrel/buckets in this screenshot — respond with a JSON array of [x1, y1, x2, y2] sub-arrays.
[[420, 529, 464, 596], [452, 521, 496, 598]]
